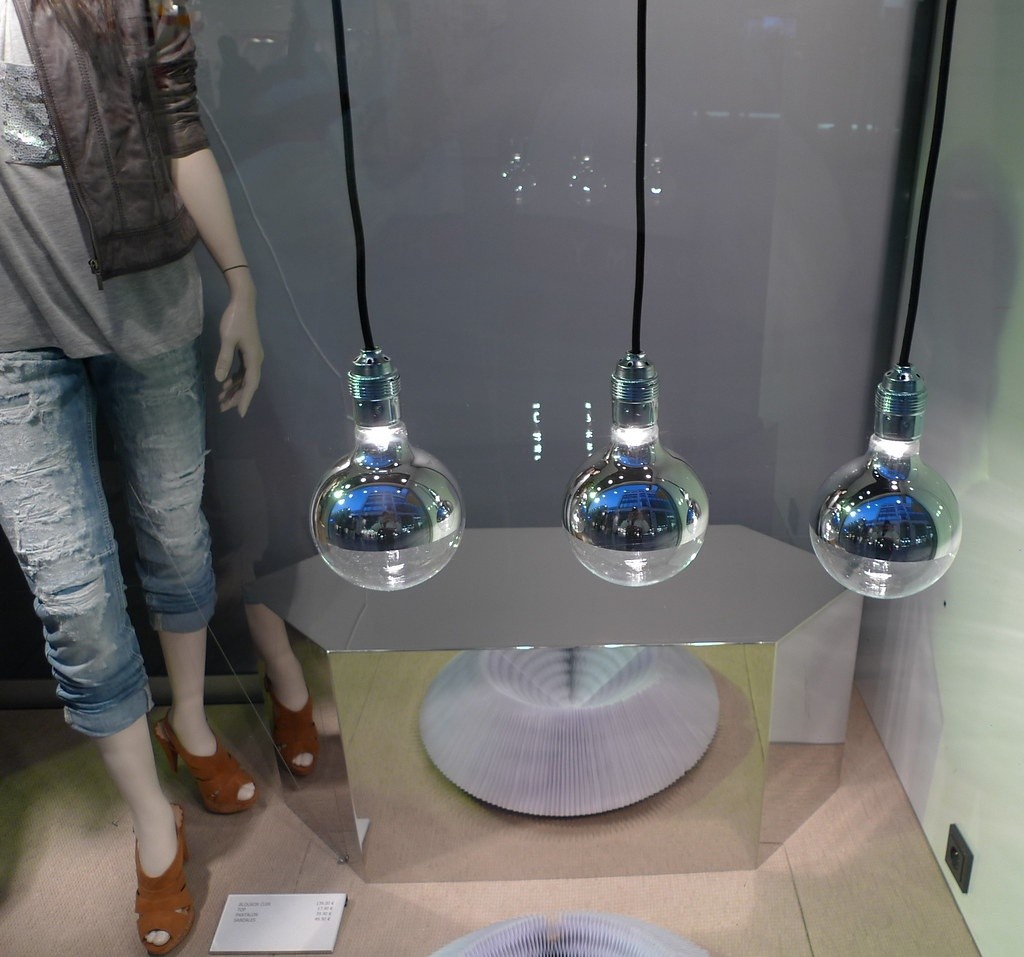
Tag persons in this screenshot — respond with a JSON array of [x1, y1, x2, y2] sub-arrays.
[[0, 0, 267, 957], [246, 601, 319, 774]]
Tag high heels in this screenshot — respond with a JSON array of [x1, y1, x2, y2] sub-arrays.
[[133, 803, 188, 957], [261, 672, 319, 774], [153, 709, 258, 815]]
